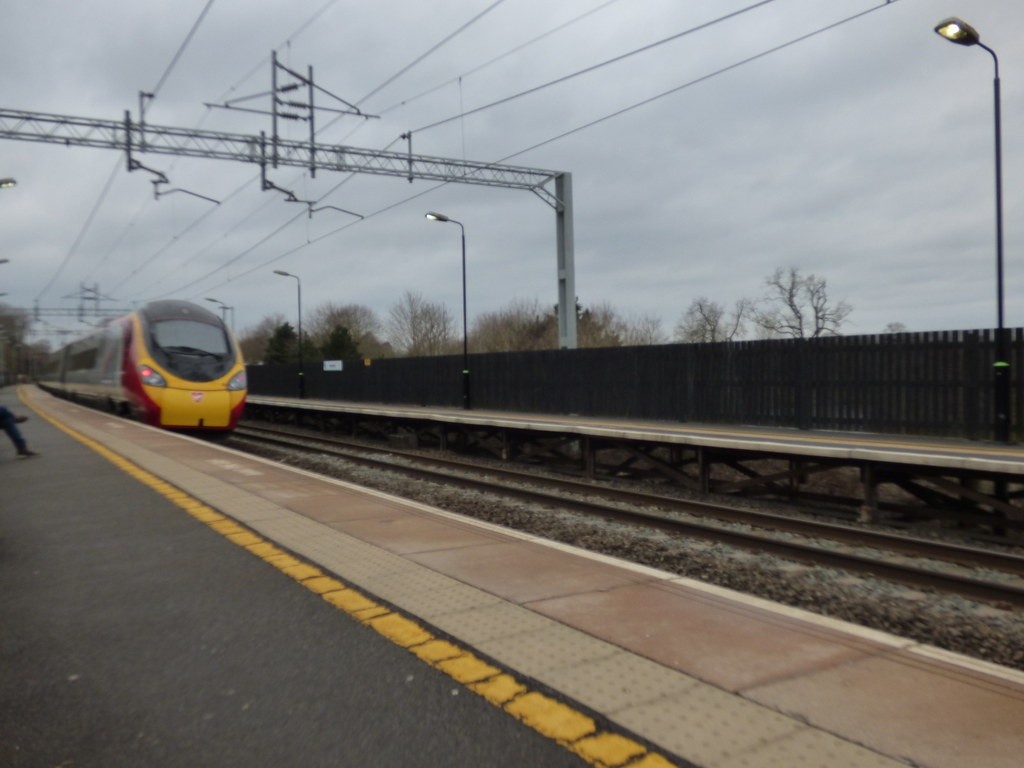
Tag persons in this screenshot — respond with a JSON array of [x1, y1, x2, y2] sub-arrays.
[[0, 405, 38, 456]]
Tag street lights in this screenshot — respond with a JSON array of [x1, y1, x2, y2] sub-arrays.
[[933, 16, 1010, 438], [271, 269, 307, 400], [424, 211, 472, 407]]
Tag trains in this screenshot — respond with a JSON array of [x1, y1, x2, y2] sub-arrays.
[[35, 297, 248, 441]]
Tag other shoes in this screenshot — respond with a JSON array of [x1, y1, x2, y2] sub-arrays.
[[14, 415, 29, 424], [17, 451, 40, 459]]
